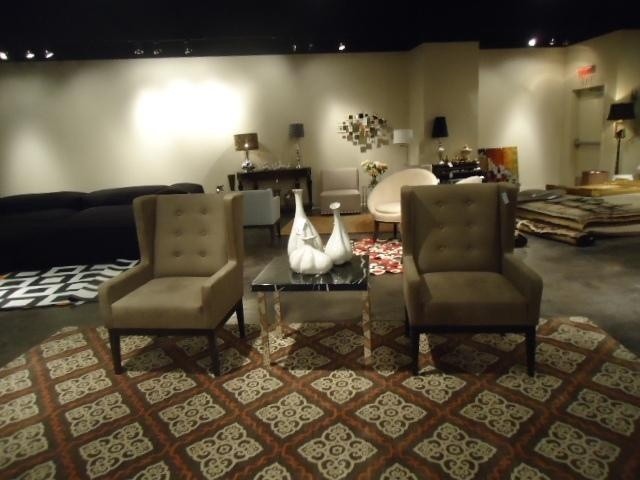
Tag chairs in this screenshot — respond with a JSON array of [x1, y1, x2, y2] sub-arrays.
[[397, 179, 544, 376], [317, 168, 361, 216], [96, 191, 247, 376], [366, 167, 440, 239], [243, 189, 283, 243]]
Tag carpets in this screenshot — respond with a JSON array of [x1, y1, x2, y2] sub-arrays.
[[352, 235, 402, 279], [280, 213, 402, 237], [0, 256, 138, 309], [1, 315, 639, 478]]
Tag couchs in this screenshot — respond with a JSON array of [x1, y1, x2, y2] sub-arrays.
[[1, 183, 207, 271]]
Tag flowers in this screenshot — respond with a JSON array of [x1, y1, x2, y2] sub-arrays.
[[360, 159, 388, 179]]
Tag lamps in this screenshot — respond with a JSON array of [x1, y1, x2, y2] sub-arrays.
[[233, 131, 262, 171], [431, 116, 448, 166], [604, 102, 636, 174], [288, 121, 306, 167], [393, 128, 413, 167]]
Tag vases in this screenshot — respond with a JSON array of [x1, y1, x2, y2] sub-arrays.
[[326, 200, 353, 265], [370, 177, 378, 185], [290, 233, 333, 276], [287, 185, 323, 252]]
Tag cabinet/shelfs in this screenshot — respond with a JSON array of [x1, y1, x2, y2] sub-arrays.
[[432, 162, 481, 184], [478, 145, 520, 180]]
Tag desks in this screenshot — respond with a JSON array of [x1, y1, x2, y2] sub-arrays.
[[250, 253, 376, 369], [237, 166, 313, 216]]
